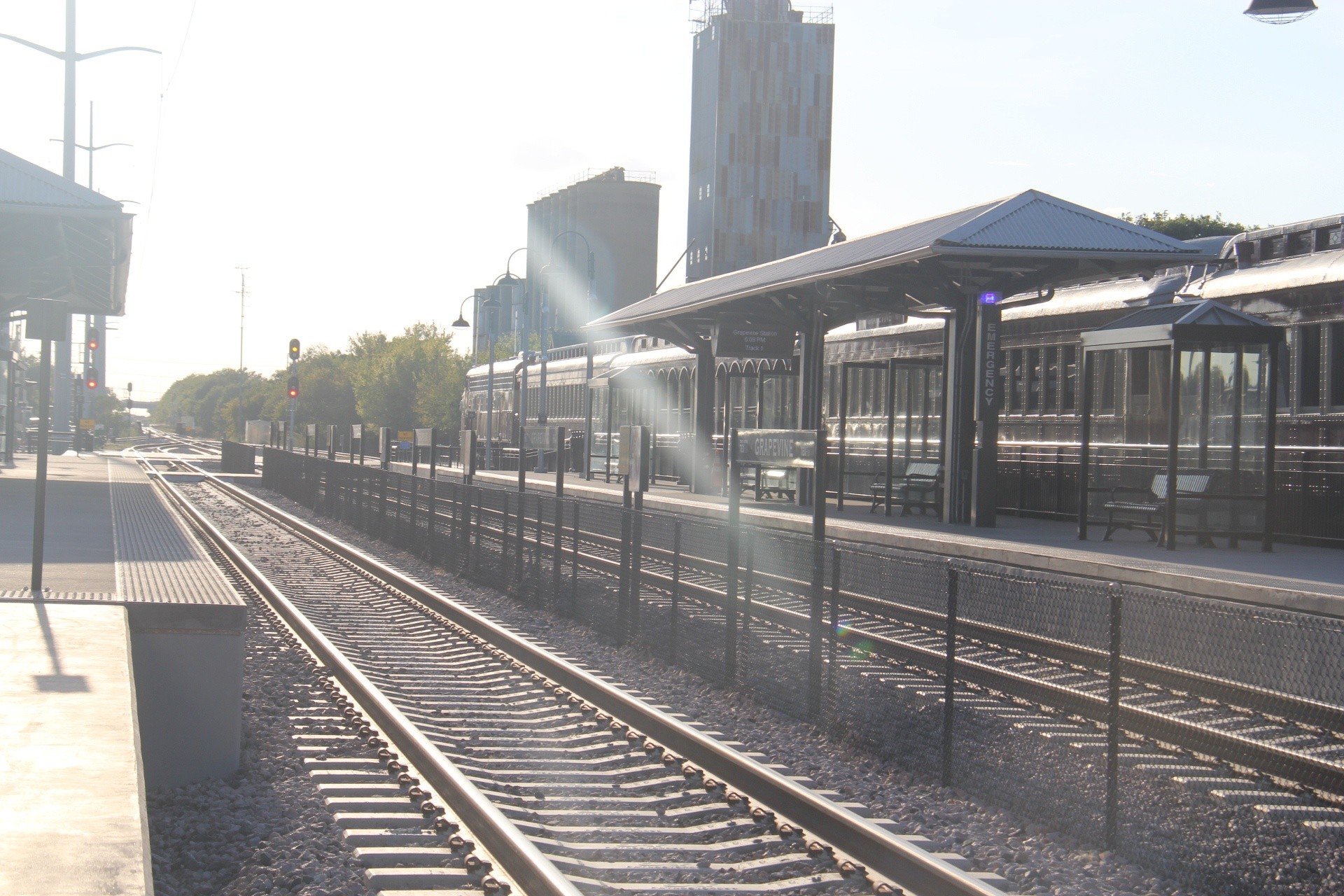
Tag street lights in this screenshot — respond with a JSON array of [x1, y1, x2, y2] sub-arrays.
[[451, 298, 496, 470], [482, 246, 553, 473], [539, 231, 594, 480]]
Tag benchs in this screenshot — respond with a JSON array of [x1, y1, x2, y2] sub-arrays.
[[870, 462, 943, 516], [1103, 468, 1223, 550]]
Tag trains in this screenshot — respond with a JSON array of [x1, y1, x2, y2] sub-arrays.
[[455, 213, 1344, 545]]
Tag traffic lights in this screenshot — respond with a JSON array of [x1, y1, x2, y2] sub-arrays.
[[88, 368, 98, 389], [287, 377, 299, 398], [289, 338, 301, 359], [88, 328, 99, 351]]
